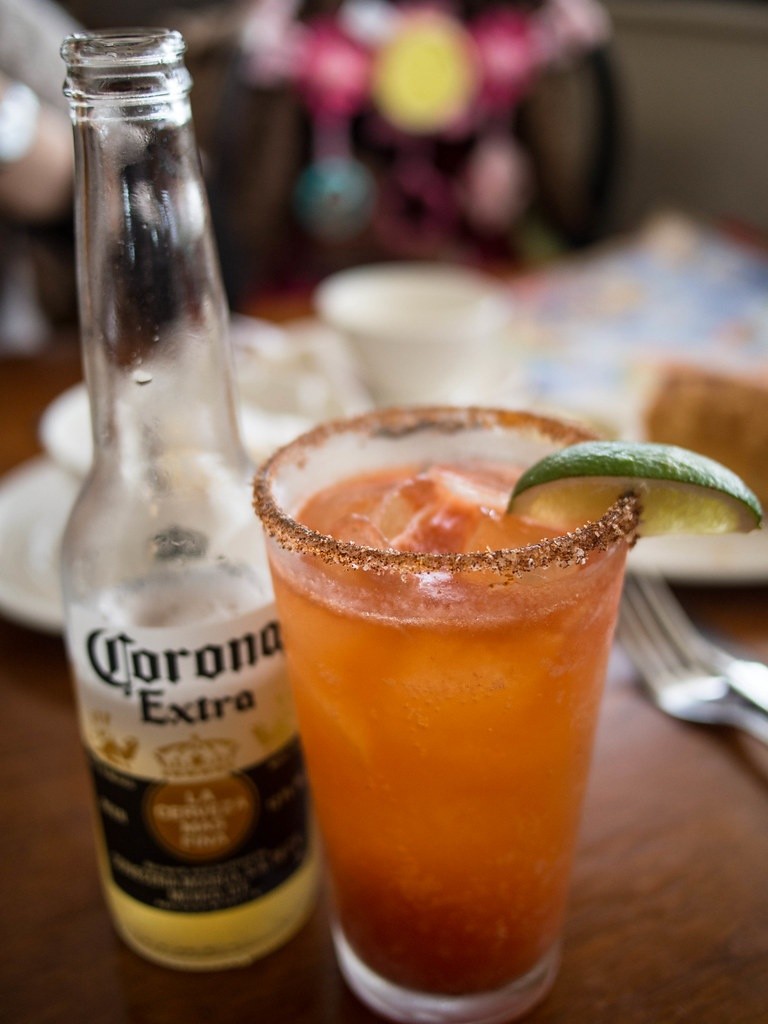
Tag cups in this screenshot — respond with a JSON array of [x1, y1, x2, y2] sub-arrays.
[[317, 265, 506, 410], [254, 398, 645, 1023]]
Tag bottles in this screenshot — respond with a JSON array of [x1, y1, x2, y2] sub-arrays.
[[52, 23, 316, 971]]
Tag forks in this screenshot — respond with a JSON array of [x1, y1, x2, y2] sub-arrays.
[[613, 567, 767, 748]]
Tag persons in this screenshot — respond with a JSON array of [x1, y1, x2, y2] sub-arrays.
[[0, 0, 123, 364]]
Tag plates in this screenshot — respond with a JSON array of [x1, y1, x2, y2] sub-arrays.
[[1, 457, 73, 635]]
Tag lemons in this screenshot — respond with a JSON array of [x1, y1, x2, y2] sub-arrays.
[[509, 442, 762, 539]]
